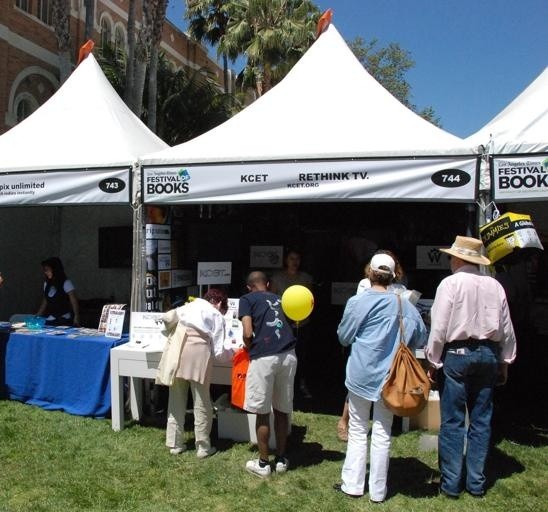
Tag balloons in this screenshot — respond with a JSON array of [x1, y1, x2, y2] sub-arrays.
[[280, 284, 314, 326]]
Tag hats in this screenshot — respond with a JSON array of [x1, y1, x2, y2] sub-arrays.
[[439, 236, 491, 266], [369, 253, 397, 278]]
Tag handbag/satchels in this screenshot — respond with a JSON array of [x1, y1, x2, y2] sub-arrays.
[[382, 342, 431, 415]]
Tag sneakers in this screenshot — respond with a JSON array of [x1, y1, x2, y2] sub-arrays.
[[333, 483, 361, 498], [246, 458, 272, 478], [170, 444, 187, 455], [335, 418, 348, 442], [274, 451, 292, 473], [196, 446, 217, 458]]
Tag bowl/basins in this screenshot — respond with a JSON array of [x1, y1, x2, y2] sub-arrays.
[[24, 316, 47, 329]]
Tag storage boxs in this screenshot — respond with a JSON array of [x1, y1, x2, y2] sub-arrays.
[[216, 407, 290, 450], [409, 398, 441, 432]]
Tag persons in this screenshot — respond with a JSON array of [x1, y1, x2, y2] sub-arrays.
[[271, 249, 312, 398], [34, 258, 79, 327], [336, 249, 406, 441], [336, 254, 428, 504], [155, 289, 228, 458], [423, 236, 517, 501], [238, 271, 297, 477]]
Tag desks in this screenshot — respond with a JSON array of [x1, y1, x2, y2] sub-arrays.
[[6, 325, 127, 418], [110, 341, 233, 432], [0, 320, 54, 364]]
